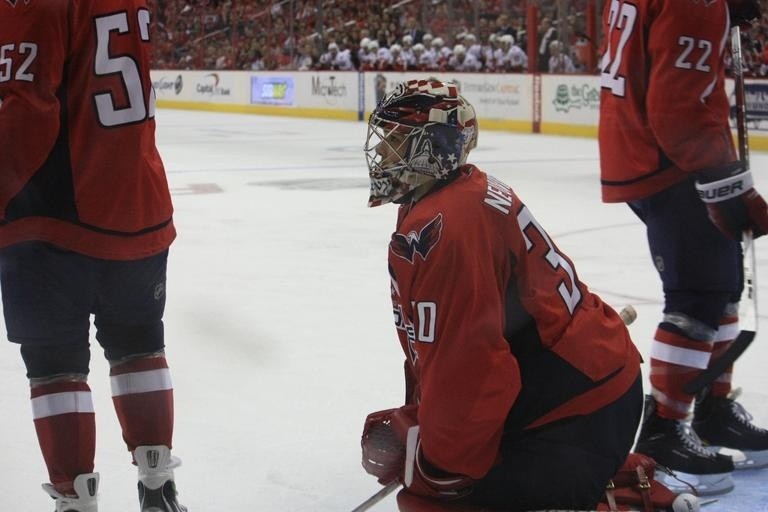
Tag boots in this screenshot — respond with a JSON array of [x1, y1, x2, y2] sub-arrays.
[[135, 445, 187, 512], [634, 394, 734, 474], [41, 473, 100, 512], [688, 382, 767, 451]]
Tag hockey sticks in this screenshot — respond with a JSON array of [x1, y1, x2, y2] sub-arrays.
[[681, 22, 758, 395]]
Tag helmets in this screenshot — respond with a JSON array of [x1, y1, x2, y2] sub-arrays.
[[328, 42, 337, 50], [360, 32, 514, 56], [551, 40, 563, 56], [363, 79, 478, 208]]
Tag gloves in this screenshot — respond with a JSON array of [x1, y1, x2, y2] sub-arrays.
[[695, 160, 768, 242], [361, 404, 475, 503]]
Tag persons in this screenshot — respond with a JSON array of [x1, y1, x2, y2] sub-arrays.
[[596, 0, 768, 495], [1, 0, 191, 512], [148, 0, 768, 78], [358, 78, 699, 512]]
